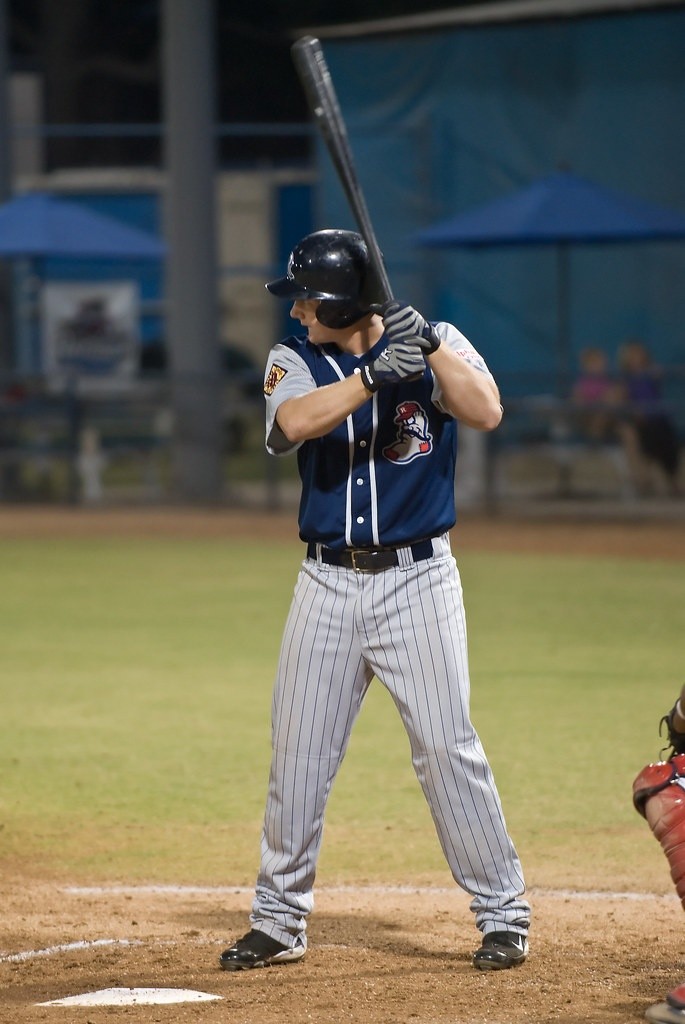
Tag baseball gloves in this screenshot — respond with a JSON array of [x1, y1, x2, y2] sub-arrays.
[[658, 683, 685, 764]]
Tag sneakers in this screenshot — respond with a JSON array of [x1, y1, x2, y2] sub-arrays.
[[219, 929, 306, 970], [473, 931, 529, 970]]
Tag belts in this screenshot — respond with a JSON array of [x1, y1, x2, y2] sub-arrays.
[[307, 540, 433, 574]]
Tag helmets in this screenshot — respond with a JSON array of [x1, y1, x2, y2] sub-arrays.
[[264, 229, 387, 329]]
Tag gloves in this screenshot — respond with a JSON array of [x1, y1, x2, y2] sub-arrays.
[[370, 300, 441, 355], [361, 343, 426, 393]]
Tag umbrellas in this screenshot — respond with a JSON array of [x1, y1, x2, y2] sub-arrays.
[[0, 189, 168, 397], [416, 165, 685, 394]]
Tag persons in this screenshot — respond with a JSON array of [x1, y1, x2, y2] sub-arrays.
[[217, 228, 530, 971], [570, 344, 685, 504], [633, 685, 685, 1024]]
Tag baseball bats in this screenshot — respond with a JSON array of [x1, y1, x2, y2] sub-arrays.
[[291, 35, 396, 306]]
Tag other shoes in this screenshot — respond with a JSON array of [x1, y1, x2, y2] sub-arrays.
[[645, 994, 685, 1024]]
